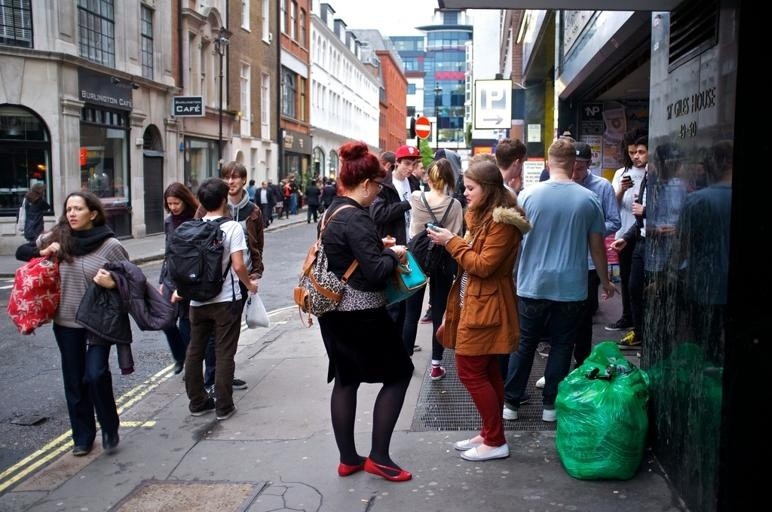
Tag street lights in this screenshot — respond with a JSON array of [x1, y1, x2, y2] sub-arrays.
[[216, 37, 228, 178], [434, 82, 442, 148]]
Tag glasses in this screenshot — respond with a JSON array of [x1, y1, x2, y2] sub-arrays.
[[363, 176, 384, 193]]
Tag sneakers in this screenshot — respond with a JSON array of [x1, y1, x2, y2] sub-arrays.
[[419, 308, 433, 324], [191, 402, 216, 416], [431, 367, 446, 381], [205, 384, 216, 402], [503, 344, 558, 424], [232, 377, 248, 390], [616, 329, 643, 350], [216, 405, 237, 420], [605, 318, 635, 331]]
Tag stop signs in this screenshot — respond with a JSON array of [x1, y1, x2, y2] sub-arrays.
[[415, 117, 431, 140]]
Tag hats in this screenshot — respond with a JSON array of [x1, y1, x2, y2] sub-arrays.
[[572, 141, 591, 162], [395, 145, 423, 158]]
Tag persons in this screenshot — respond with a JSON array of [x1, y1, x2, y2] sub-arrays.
[[24, 181, 50, 244], [247, 174, 338, 230], [376, 145, 495, 380], [158, 165, 265, 420], [16, 191, 130, 456], [427, 163, 533, 463], [494, 137, 646, 423], [315, 140, 415, 482]]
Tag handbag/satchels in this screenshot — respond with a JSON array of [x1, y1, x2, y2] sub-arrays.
[[6, 255, 60, 335], [380, 249, 428, 308], [293, 205, 359, 318], [15, 197, 26, 235]]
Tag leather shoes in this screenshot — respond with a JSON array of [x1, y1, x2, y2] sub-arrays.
[[72, 443, 91, 456], [460, 444, 510, 461], [364, 458, 412, 482], [175, 362, 183, 373], [102, 430, 119, 450], [339, 463, 364, 476], [454, 439, 482, 451]]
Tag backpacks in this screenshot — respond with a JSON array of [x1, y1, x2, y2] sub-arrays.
[[406, 192, 455, 276], [166, 216, 233, 303]]
